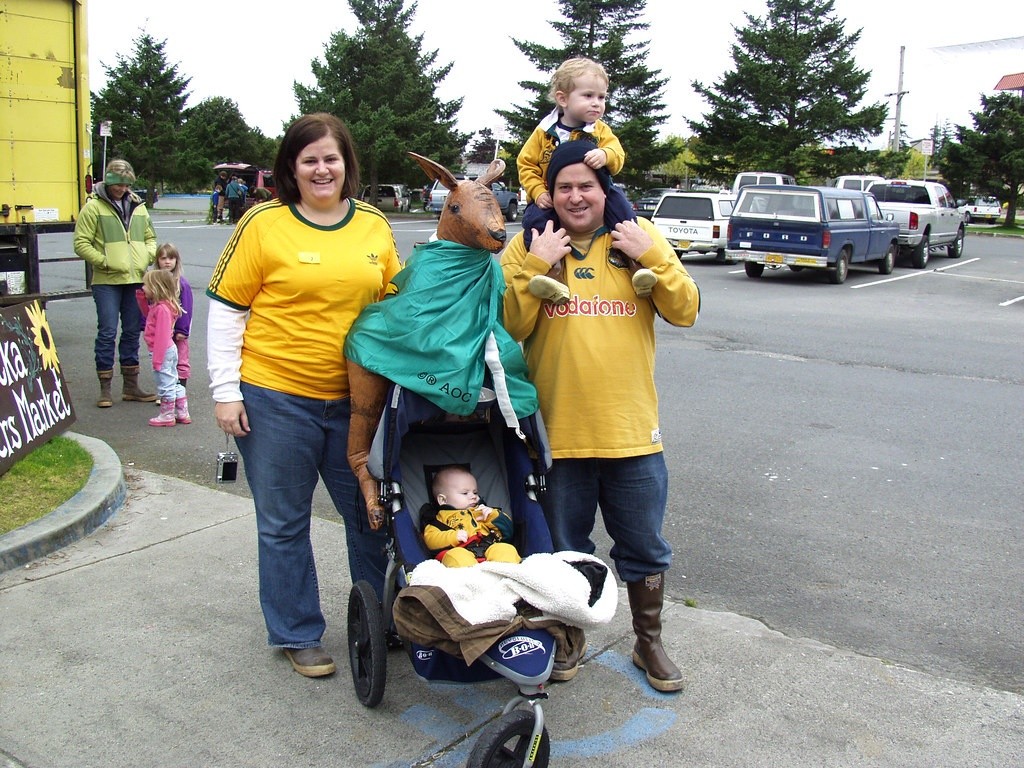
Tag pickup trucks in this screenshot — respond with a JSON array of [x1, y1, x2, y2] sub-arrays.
[[726, 183, 899, 285], [866, 179, 965, 269]]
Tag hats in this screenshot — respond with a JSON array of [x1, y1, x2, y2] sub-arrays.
[[546, 140, 611, 202], [106, 160, 136, 186]]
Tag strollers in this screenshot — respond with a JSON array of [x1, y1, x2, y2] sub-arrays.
[[347, 376, 570, 768]]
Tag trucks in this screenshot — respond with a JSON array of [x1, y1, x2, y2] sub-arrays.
[[0, 0, 95, 307]]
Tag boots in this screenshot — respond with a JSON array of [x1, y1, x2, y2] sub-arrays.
[[550, 626, 587, 681], [528, 257, 570, 305], [175, 397, 192, 424], [120, 366, 157, 401], [626, 571, 684, 690], [148, 402, 176, 427], [97, 369, 113, 406], [625, 253, 657, 298]]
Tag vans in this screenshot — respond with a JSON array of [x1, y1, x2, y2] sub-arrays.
[[733, 172, 796, 193], [829, 176, 885, 210], [650, 192, 738, 264]]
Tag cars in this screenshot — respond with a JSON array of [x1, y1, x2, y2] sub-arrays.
[[632, 189, 685, 218], [135, 188, 158, 206]]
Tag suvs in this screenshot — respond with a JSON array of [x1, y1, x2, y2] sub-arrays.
[[957, 196, 1001, 224], [421, 185, 431, 212], [212, 162, 277, 216], [362, 185, 410, 213], [518, 185, 530, 215], [425, 173, 519, 222]]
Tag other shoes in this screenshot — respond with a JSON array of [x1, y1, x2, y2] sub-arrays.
[[283, 646, 336, 677]]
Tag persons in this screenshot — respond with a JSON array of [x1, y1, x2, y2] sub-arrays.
[[496, 139, 701, 693], [207, 113, 405, 678], [421, 468, 523, 568], [249, 186, 273, 204], [72, 159, 157, 408], [155, 242, 194, 405], [516, 56, 658, 303], [134, 269, 192, 427], [209, 171, 248, 225]]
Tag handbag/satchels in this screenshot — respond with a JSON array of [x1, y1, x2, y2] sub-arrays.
[[239, 195, 244, 207]]
